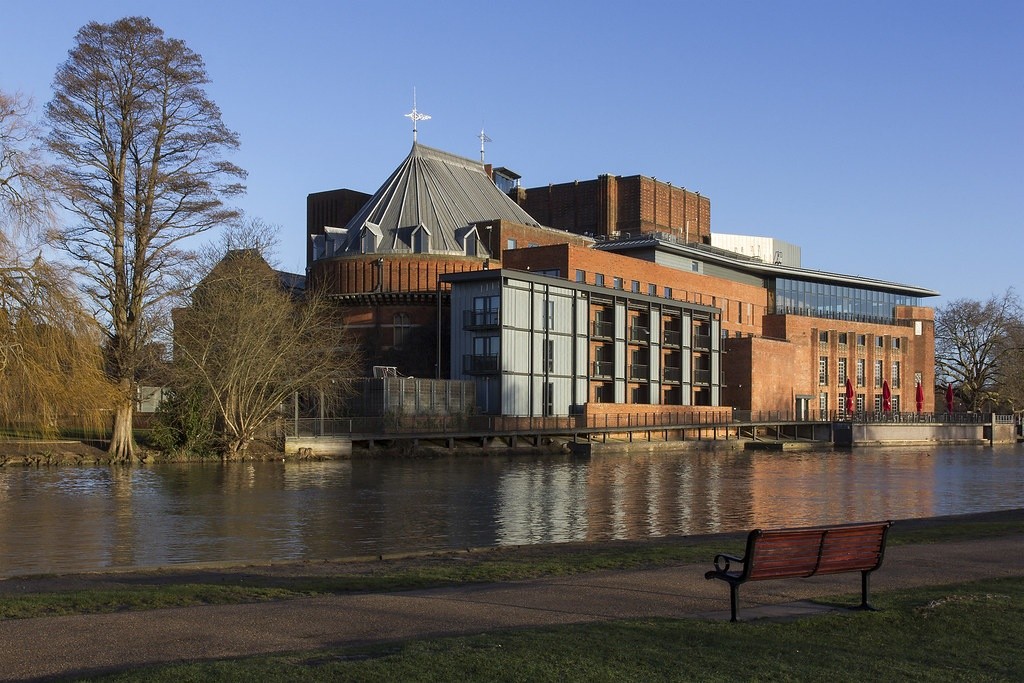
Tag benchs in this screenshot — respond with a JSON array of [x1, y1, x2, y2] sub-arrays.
[[705, 520, 897, 624]]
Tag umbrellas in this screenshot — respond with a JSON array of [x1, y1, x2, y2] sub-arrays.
[[845, 378, 852, 416], [946, 383, 955, 414], [883, 380, 891, 413], [916, 380, 924, 415]]
